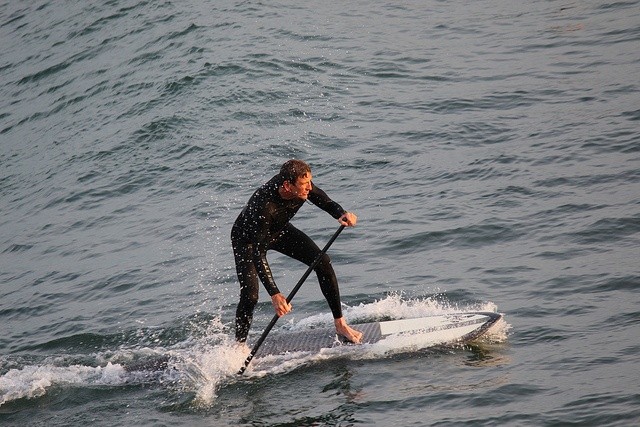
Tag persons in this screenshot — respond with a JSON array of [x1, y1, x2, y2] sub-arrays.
[[230, 160, 363, 345]]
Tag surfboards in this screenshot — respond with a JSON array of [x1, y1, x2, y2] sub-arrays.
[[84, 312, 501, 383]]
